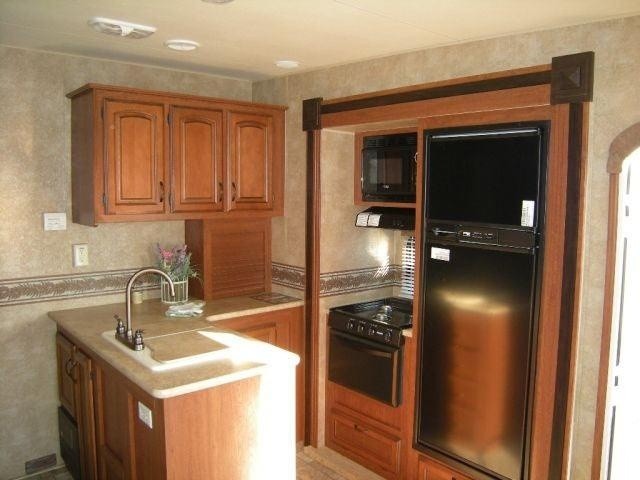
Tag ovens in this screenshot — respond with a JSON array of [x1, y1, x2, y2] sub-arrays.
[[325, 330, 401, 410]]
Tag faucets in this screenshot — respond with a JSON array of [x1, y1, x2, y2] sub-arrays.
[[125, 267, 175, 344]]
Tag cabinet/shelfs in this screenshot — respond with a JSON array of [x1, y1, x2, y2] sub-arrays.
[[65, 84, 167, 227], [169, 94, 287, 222], [213, 305, 311, 452], [324, 378, 411, 480], [53, 327, 90, 480], [90, 361, 139, 479], [408, 448, 467, 479]]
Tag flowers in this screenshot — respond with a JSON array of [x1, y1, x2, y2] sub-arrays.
[[157, 241, 202, 287]]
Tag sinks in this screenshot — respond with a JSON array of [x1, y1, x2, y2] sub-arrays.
[[140, 329, 237, 373]]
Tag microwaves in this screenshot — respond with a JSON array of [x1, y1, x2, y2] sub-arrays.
[[358, 135, 416, 201]]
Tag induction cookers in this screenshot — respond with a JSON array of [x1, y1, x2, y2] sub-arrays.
[[327, 294, 415, 345]]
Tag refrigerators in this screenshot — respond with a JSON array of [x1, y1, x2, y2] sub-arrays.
[[416, 126, 542, 480]]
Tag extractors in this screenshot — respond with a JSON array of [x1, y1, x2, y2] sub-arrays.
[[355, 206, 416, 232]]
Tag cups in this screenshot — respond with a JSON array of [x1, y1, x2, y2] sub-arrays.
[[131, 291, 143, 303]]
